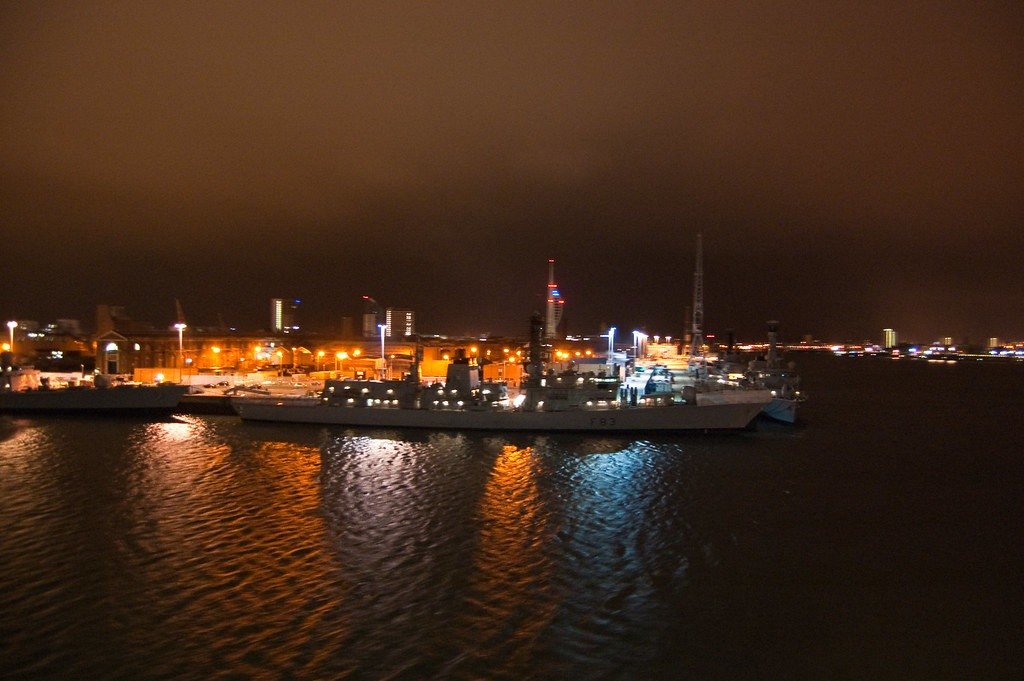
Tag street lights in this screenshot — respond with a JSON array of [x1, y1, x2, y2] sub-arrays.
[[174, 323, 187, 382], [7, 320, 18, 351]]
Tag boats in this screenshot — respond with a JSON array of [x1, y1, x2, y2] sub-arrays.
[[0, 349, 191, 407], [739, 319, 807, 424], [221, 315, 775, 435]]
